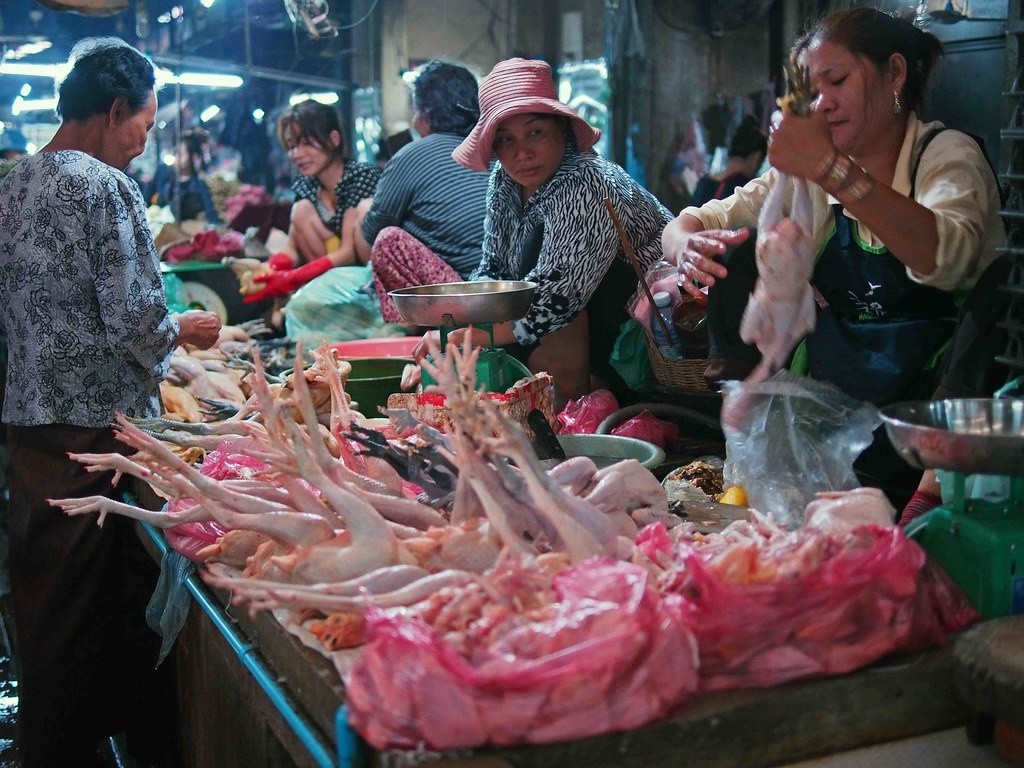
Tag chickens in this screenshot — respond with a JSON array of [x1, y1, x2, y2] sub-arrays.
[[221, 255, 277, 296], [718, 55, 830, 439], [54, 324, 946, 752]]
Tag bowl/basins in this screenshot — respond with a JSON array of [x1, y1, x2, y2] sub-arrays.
[[310, 336, 428, 359], [281, 358, 421, 420], [524, 427, 666, 488]]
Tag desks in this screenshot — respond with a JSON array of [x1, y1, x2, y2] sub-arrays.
[[122, 464, 1002, 768]]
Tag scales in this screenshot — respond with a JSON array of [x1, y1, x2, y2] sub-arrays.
[[875, 395, 1024, 628], [386, 280, 540, 398], [156, 258, 230, 329]]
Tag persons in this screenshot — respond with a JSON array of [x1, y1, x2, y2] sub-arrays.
[[241, 101, 381, 329], [147, 126, 221, 224], [0, 131, 28, 178], [662, 4, 1007, 531], [396, 58, 696, 406], [356, 65, 497, 336], [687, 111, 770, 208], [0, 37, 226, 766]]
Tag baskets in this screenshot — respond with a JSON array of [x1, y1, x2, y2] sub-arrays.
[[644, 330, 709, 392]]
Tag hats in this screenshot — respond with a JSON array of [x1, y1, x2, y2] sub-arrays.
[[450, 58, 601, 170]]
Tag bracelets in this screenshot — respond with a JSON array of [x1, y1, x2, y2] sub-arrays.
[[808, 151, 873, 204]]
